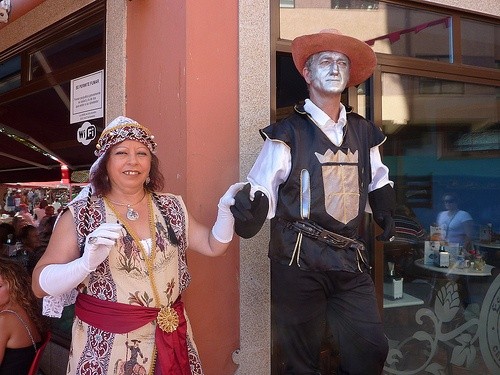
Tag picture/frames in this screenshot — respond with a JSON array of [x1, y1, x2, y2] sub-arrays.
[[436, 127, 500, 161]]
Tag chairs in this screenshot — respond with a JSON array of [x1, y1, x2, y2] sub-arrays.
[[383, 241, 424, 309], [28, 329, 52, 375]]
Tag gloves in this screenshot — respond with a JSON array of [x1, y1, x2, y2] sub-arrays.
[[229, 180, 270, 239], [366, 184, 398, 241], [210, 182, 251, 243], [39, 223, 127, 297]]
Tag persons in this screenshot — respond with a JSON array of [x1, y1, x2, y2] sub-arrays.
[[0, 259, 46, 375], [388, 204, 433, 325], [0, 188, 82, 295], [436, 190, 474, 323], [229, 37, 399, 375], [31, 114, 255, 375]]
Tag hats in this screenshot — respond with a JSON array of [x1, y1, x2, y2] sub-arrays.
[[291, 29, 377, 87], [88, 116, 159, 183]]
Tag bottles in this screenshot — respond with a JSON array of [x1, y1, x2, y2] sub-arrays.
[[479, 223, 495, 244], [439, 246, 446, 253], [388, 269, 395, 281], [474, 255, 484, 271]]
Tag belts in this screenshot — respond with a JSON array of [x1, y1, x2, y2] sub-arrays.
[[276, 217, 362, 250]]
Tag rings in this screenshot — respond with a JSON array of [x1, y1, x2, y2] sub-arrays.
[[389, 235, 395, 242], [87, 237, 98, 244]]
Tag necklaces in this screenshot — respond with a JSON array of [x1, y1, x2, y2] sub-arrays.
[[106, 188, 146, 221], [444, 209, 459, 238]]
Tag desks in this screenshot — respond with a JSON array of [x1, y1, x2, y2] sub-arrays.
[[415, 258, 496, 307], [473, 243, 500, 284]]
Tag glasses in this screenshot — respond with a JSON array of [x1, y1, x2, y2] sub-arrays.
[[443, 198, 458, 204]]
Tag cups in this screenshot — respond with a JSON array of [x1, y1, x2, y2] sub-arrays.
[[457, 255, 470, 269]]
